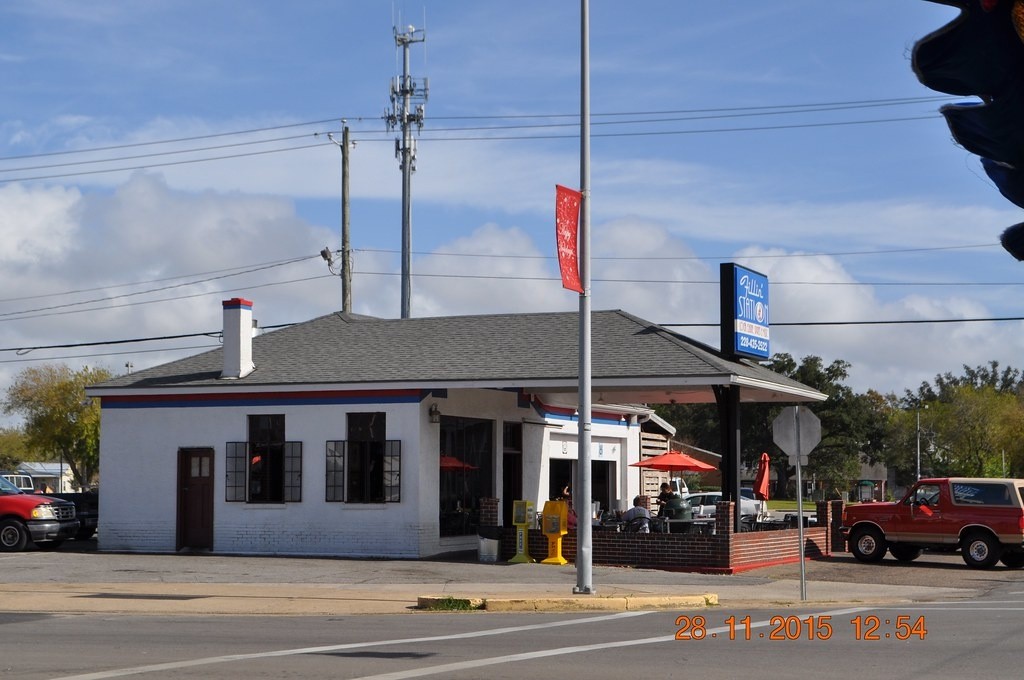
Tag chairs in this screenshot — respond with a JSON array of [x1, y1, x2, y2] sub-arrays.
[[569, 502, 811, 540]]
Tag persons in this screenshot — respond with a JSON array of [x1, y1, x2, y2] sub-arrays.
[[655, 482, 676, 521], [619, 494, 655, 535], [563, 479, 573, 505]]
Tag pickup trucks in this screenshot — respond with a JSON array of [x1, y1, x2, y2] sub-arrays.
[[1, 469, 98, 555]]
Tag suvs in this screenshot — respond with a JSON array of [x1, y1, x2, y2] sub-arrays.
[[838, 478, 1024, 570]]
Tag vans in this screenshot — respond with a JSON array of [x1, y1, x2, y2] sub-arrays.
[[669, 477, 689, 499]]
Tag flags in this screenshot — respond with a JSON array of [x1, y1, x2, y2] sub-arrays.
[[554, 183, 586, 293]]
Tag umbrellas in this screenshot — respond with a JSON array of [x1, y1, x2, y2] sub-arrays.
[[439, 456, 480, 514], [627, 449, 719, 495], [752, 452, 771, 531]]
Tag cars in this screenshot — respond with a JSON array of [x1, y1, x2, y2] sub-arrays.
[[682, 487, 768, 523]]
